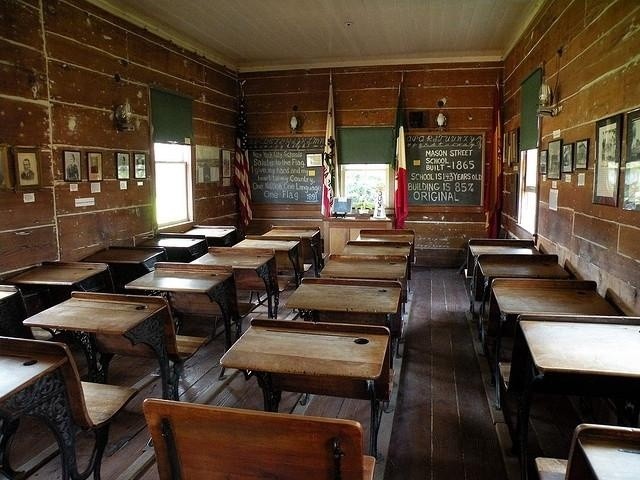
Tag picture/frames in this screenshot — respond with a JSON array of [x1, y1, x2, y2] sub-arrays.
[[625, 107, 640, 164], [133, 152, 147, 179], [62, 149, 82, 182], [86, 151, 104, 183], [505, 128, 519, 167], [13, 147, 42, 193], [575, 138, 590, 170], [115, 151, 131, 180], [539, 138, 575, 180], [305, 153, 324, 168], [221, 149, 232, 178]]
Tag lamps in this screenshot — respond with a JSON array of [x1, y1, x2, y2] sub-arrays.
[[434, 100, 447, 132], [536, 75, 559, 118], [290, 105, 298, 130], [118, 96, 137, 132]]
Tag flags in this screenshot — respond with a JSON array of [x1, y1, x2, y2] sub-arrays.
[[393, 81, 409, 229], [233, 78, 253, 237], [319, 79, 340, 217], [484, 71, 503, 237]]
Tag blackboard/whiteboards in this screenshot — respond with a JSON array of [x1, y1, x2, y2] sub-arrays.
[[247, 131, 486, 208]]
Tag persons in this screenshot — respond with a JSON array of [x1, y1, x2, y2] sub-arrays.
[[21, 158, 34, 180], [67, 154, 79, 180]]
